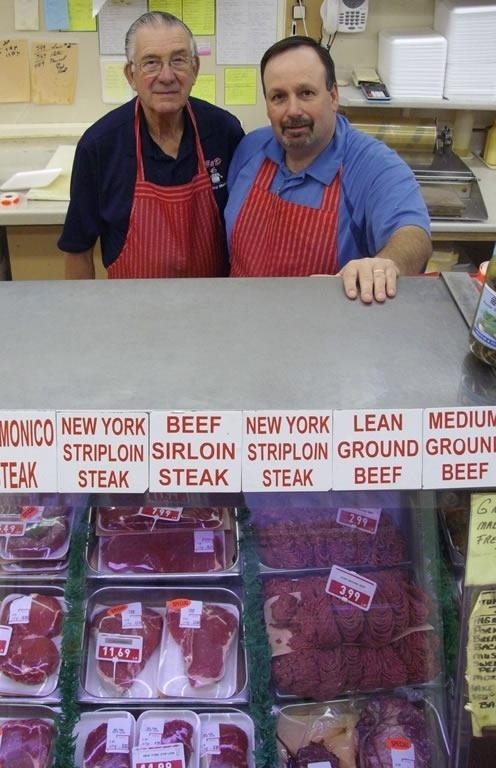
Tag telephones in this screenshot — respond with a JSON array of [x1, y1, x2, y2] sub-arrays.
[[322, 2, 368, 34]]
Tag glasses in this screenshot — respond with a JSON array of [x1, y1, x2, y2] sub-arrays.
[[129, 55, 196, 76]]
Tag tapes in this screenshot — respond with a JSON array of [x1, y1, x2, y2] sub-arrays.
[[1, 193, 21, 207]]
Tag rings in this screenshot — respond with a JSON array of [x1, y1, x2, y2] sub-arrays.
[[373, 269, 384, 273]]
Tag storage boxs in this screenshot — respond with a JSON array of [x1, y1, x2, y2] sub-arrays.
[[0, 277, 496, 768]]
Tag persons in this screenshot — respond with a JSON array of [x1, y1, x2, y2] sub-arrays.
[[224, 35, 433, 302], [57, 11, 245, 278]]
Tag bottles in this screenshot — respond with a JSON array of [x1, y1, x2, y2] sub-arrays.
[[466, 248, 496, 370], [451, 109, 476, 157]]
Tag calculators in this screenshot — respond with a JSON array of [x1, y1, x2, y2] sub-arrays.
[[361, 82, 391, 100]]
